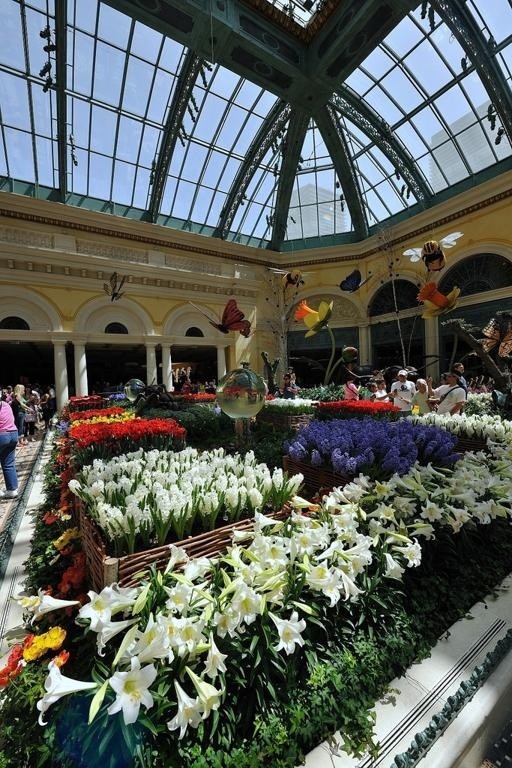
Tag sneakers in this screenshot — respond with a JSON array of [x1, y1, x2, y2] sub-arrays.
[[1, 491, 18, 499]]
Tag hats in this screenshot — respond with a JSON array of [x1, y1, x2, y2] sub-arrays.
[[398, 370, 408, 377], [445, 372, 460, 380]]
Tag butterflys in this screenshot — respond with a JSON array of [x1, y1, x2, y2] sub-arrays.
[[339, 269, 373, 294], [104, 271, 125, 302], [209, 298, 251, 338], [477, 317, 512, 358]]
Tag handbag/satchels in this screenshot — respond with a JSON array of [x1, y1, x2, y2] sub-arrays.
[[25, 413, 36, 422]]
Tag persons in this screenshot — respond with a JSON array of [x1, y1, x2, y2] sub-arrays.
[[0, 384, 56, 499], [343, 362, 469, 418], [287, 366, 296, 384], [279, 373, 298, 399]]
